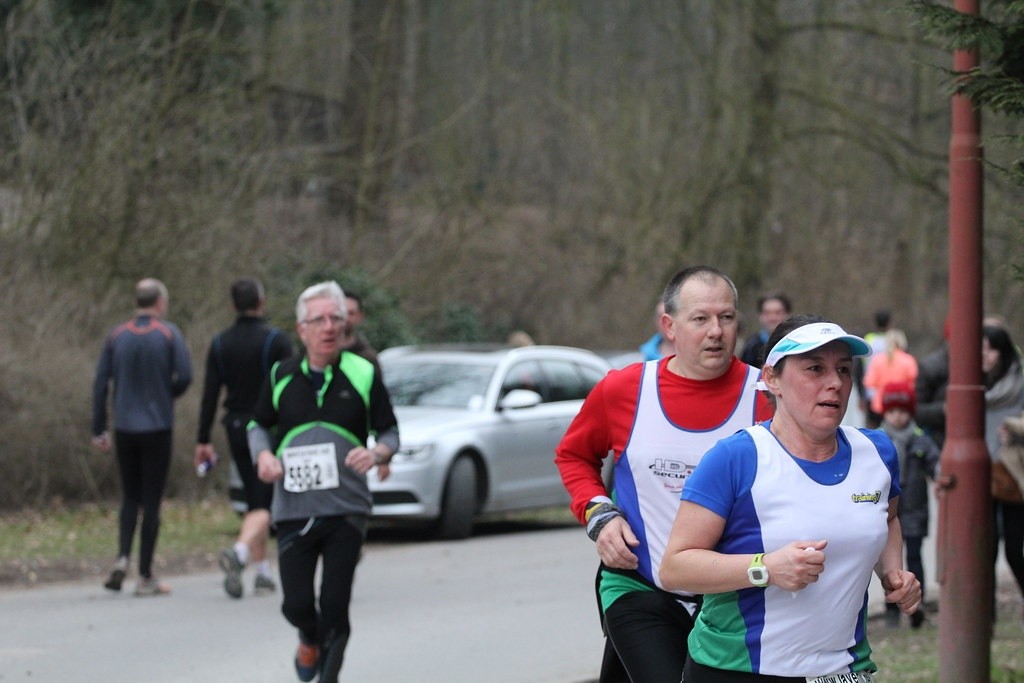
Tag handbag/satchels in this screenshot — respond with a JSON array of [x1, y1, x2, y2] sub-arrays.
[[990, 460, 1023, 500]]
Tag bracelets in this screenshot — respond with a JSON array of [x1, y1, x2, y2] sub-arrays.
[[373, 451, 382, 464]]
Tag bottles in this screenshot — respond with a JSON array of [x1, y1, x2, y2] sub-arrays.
[[195, 453, 222, 477]]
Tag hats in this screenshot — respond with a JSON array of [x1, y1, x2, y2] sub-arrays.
[[756, 321, 872, 381], [880, 384, 916, 416]]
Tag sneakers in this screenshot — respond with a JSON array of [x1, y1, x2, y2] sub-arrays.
[[295, 641, 320, 682]]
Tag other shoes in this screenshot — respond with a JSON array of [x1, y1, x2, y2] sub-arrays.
[[219, 548, 244, 598], [887, 602, 899, 615], [253, 574, 275, 592], [137, 577, 172, 595], [104, 563, 127, 591], [910, 609, 924, 628]]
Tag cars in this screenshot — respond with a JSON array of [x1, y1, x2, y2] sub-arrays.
[[609, 354, 870, 433], [366, 340, 615, 539]]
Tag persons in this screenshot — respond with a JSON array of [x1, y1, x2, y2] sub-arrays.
[[92, 278, 192, 596], [740, 293, 792, 370], [640, 302, 675, 361], [658, 314, 922, 683], [854, 310, 950, 629], [246, 281, 399, 683], [340, 292, 390, 480], [196, 279, 292, 599], [555, 265, 776, 683], [982, 317, 1024, 598]]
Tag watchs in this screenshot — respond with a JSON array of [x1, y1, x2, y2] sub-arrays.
[[748, 553, 769, 587]]
